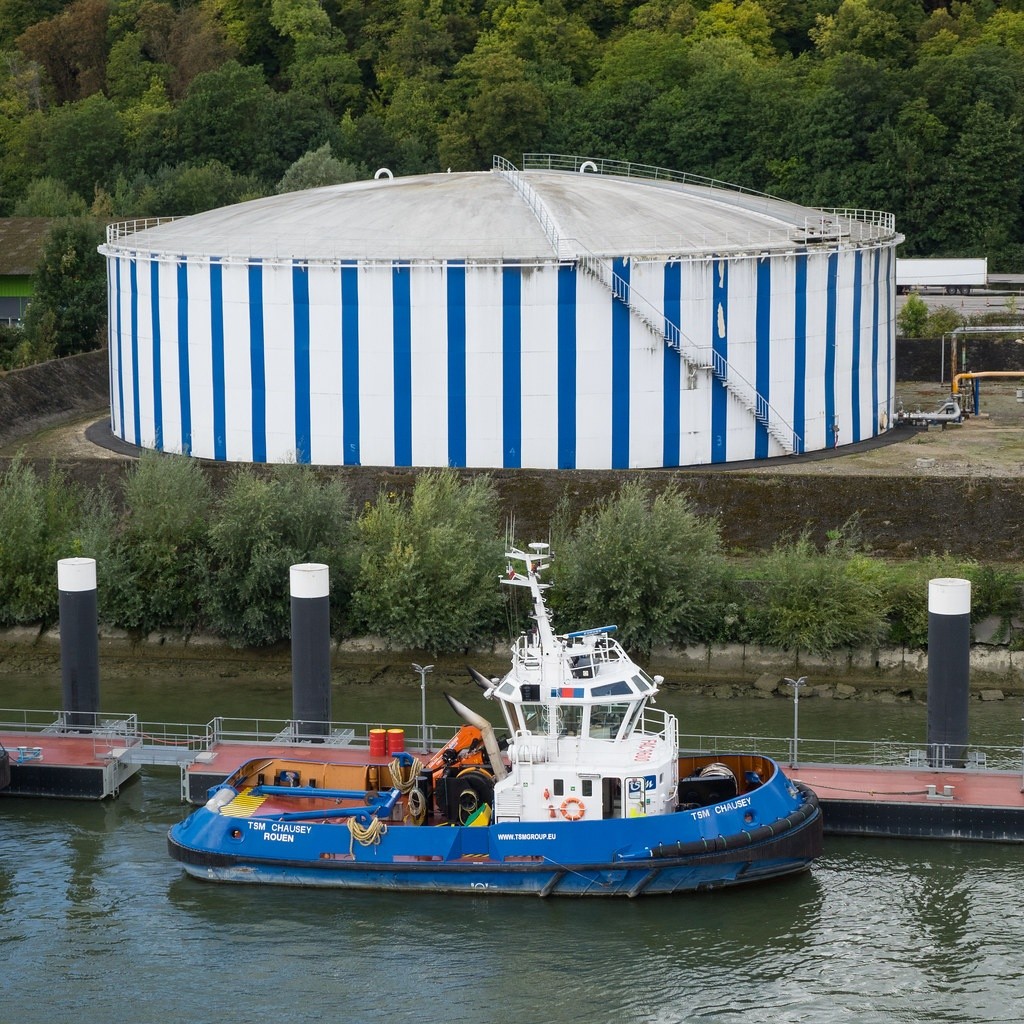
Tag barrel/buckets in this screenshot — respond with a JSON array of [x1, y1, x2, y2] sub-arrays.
[[370, 728, 386, 756], [387, 728, 404, 757]]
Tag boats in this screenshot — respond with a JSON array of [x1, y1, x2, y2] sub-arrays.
[[166, 540, 826, 898]]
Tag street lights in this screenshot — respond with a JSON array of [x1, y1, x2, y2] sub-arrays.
[[784, 676, 810, 770], [411, 662, 435, 755]]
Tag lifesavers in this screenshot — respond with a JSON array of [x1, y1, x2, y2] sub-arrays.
[[561, 797, 586, 821]]
